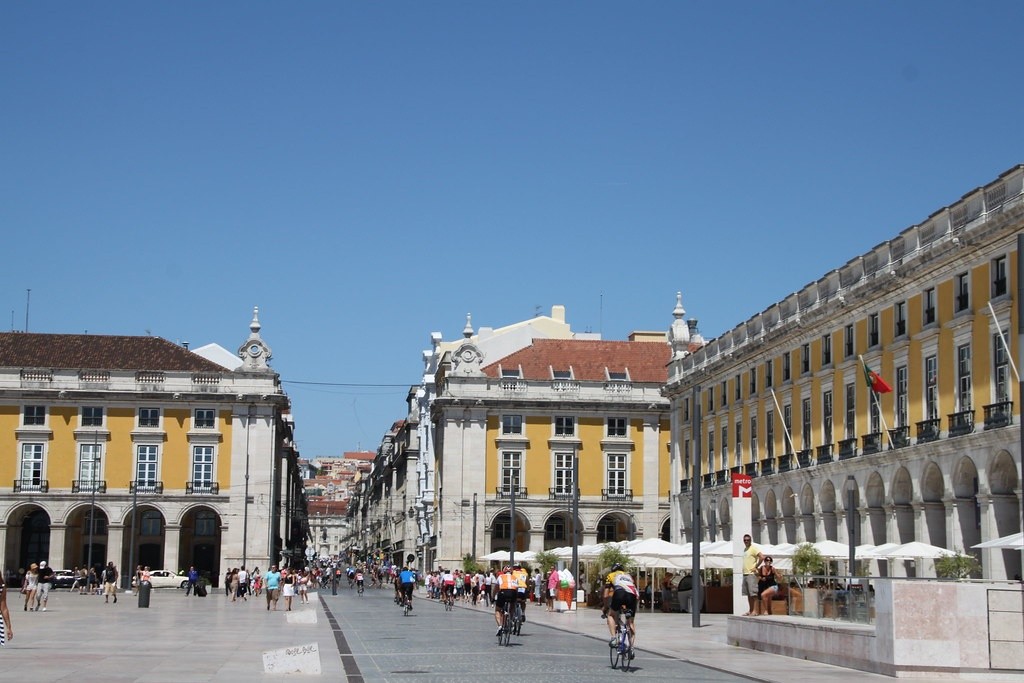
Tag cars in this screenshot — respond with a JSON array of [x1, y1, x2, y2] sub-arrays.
[[132, 570, 193, 589]]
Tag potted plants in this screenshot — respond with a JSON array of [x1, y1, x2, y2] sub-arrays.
[[202, 577, 212, 594]]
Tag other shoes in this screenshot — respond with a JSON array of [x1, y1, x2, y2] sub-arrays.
[[35, 604, 40, 611], [113, 598, 117, 603], [307, 601, 309, 603], [104, 601, 108, 604], [751, 612, 760, 615], [629, 647, 634, 660], [521, 613, 526, 622], [301, 601, 303, 603], [30, 607, 33, 611], [495, 626, 503, 636], [42, 607, 46, 611], [24, 605, 27, 611], [410, 605, 413, 611], [609, 638, 618, 647], [400, 601, 404, 606], [743, 612, 751, 615]]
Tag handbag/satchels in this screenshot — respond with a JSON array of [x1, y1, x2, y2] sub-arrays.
[[481, 584, 486, 590], [306, 579, 312, 587], [20, 586, 27, 594]]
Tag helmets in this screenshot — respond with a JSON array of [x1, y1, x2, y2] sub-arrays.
[[513, 563, 521, 568], [611, 562, 624, 571], [445, 569, 450, 573], [502, 565, 512, 574]]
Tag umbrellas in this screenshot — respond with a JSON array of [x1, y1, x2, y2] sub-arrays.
[[479, 531, 1024, 610]]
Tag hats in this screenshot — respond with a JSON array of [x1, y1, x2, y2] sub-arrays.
[[40, 561, 47, 569], [272, 565, 278, 569], [31, 563, 38, 570]]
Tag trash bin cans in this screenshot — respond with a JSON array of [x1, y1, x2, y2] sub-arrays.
[[122, 575, 129, 589], [137, 580, 151, 608]]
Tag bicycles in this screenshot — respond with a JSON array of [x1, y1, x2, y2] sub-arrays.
[[345, 573, 365, 594], [601, 608, 635, 672], [390, 582, 414, 617], [490, 593, 523, 647], [441, 586, 454, 611]]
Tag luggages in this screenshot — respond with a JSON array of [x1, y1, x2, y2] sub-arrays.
[[195, 580, 208, 597]]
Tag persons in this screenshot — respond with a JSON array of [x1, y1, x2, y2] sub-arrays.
[[70, 562, 118, 604], [185, 566, 198, 597], [742, 534, 764, 616], [492, 562, 527, 635], [225, 557, 403, 611], [534, 568, 542, 606], [24, 561, 55, 611], [548, 565, 559, 609], [0, 572, 14, 646], [134, 565, 151, 596], [398, 567, 415, 610], [545, 571, 553, 611], [602, 565, 640, 659], [411, 565, 505, 607], [750, 557, 783, 615]]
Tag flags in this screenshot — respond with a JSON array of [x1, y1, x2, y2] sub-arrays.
[[864, 364, 892, 393]]
